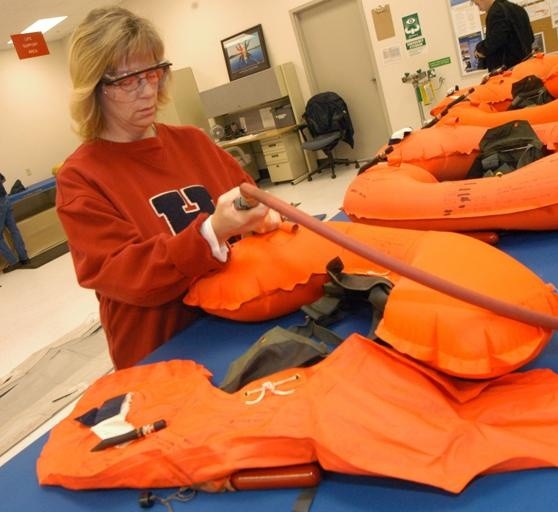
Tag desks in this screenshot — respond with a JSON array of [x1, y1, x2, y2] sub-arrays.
[[0, 53, 558, 512]]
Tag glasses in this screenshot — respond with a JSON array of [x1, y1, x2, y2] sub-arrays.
[[99, 59, 173, 103]]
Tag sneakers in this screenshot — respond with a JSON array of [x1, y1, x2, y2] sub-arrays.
[[2, 258, 31, 273]]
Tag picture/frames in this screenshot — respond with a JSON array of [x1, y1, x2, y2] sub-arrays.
[[220, 25, 270, 82]]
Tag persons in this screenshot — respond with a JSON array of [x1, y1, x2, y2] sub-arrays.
[[55, 4, 282, 372], [472, 0, 535, 75]]
